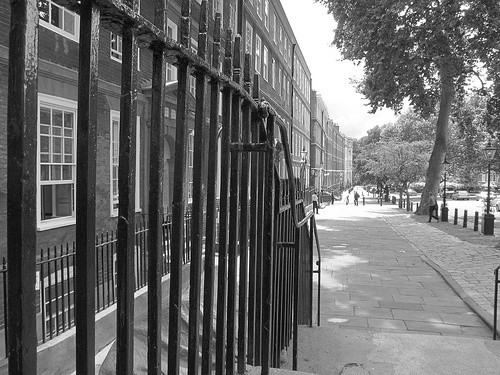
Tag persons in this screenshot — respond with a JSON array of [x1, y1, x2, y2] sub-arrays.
[[354, 191, 360, 206], [425, 192, 439, 223], [312, 192, 318, 214]]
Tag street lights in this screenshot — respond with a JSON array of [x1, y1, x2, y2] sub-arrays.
[[481, 138, 498, 236], [441, 156, 451, 222]]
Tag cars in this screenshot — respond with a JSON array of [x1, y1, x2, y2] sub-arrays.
[[404, 189, 417, 196], [476, 192, 496, 202], [442, 190, 454, 198], [496, 199, 500, 212], [452, 190, 469, 200]]
